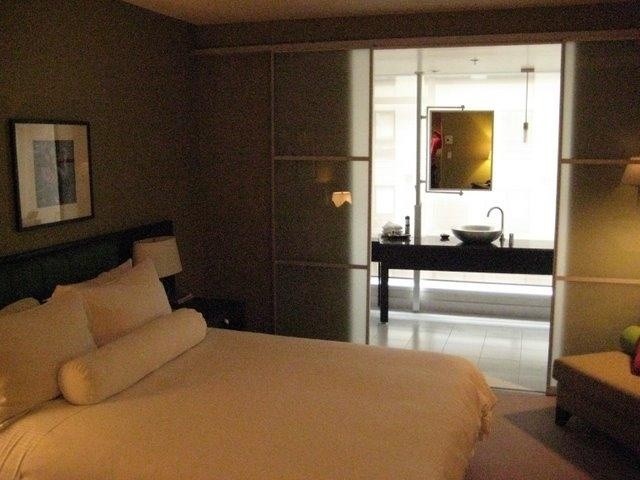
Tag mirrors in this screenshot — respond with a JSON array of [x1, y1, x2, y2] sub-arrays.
[[430, 111, 493, 192]]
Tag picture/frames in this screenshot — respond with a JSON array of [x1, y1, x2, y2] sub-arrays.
[[9, 118, 95, 233]]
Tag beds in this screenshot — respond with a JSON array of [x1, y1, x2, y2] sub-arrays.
[[1, 220, 496, 479]]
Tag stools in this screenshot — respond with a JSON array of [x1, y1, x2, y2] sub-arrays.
[[550, 350, 640, 461]]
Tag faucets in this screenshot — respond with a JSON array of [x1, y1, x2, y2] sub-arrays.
[[487, 207, 506, 242]]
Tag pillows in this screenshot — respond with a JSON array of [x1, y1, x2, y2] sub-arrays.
[[0, 259, 205, 423]]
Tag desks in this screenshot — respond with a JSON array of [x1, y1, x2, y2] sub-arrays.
[[371, 240, 556, 326]]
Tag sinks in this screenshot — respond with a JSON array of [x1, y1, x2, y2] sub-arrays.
[[452, 225, 502, 244]]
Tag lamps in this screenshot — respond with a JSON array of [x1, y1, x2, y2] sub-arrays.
[[521, 66, 536, 144], [132, 236, 184, 314]]
